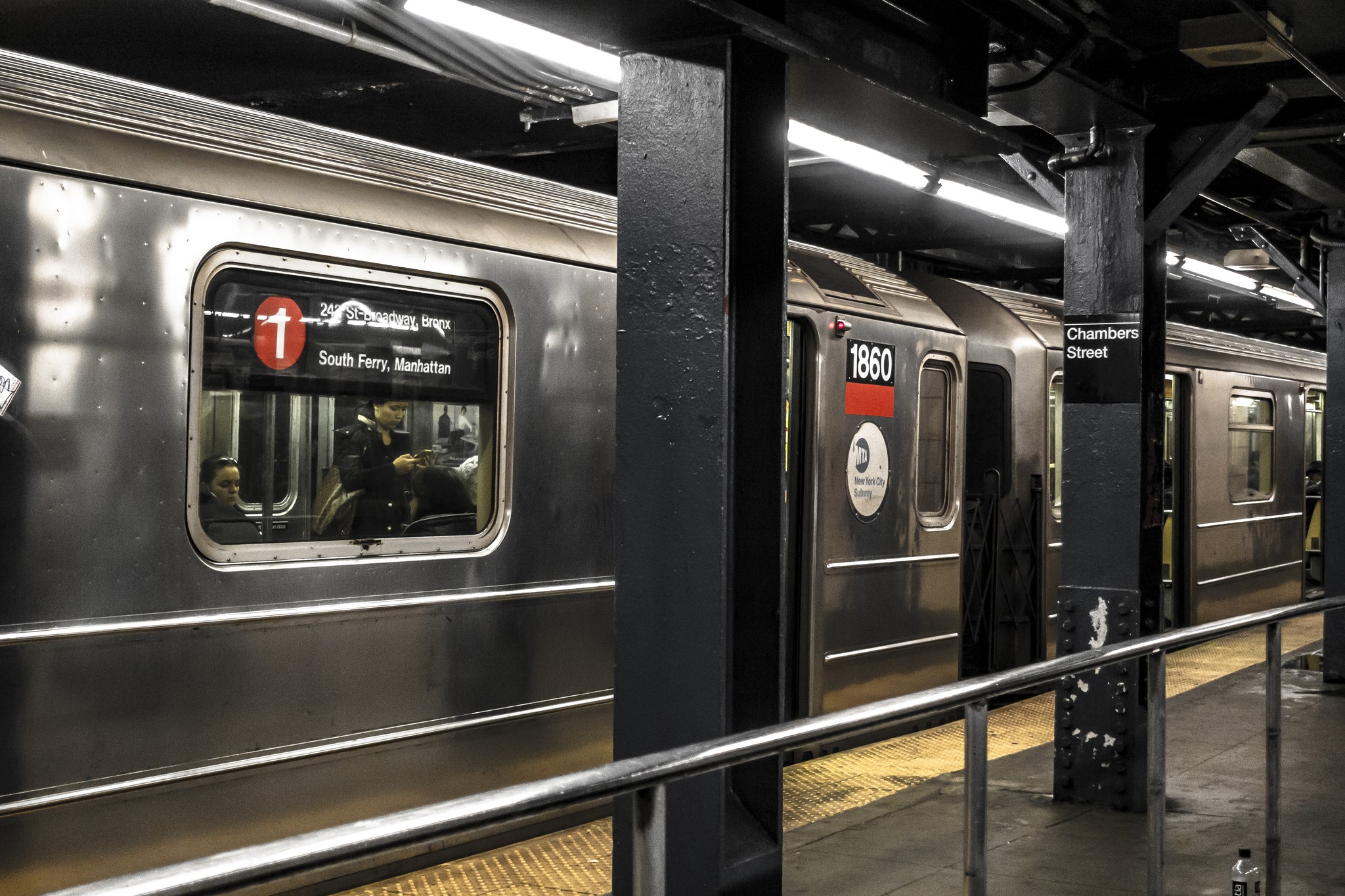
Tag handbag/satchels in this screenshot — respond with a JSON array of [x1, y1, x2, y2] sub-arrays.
[[311, 463, 366, 541]]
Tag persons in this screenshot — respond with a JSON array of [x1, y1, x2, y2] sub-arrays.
[[409, 465, 473, 521], [1247, 451, 1260, 496], [1306, 461, 1322, 540], [198, 455, 260, 533], [438, 405, 450, 440], [457, 405, 473, 436], [338, 395, 430, 538]]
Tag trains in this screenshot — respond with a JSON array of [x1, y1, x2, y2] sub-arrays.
[[0, 49, 1331, 893]]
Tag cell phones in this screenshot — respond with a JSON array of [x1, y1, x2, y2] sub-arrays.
[[413, 449, 432, 459]]
